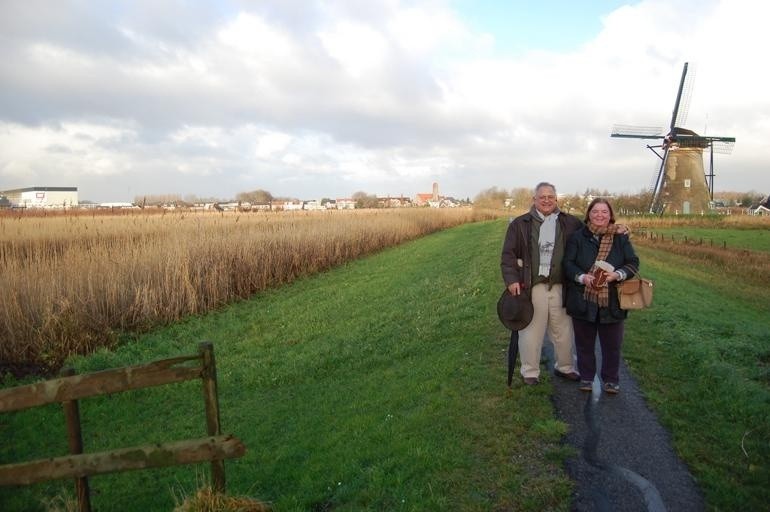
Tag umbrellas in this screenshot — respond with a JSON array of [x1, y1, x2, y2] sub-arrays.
[[505, 328, 521, 390]]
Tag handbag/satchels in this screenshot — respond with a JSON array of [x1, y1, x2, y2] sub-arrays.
[[616, 264, 653, 310]]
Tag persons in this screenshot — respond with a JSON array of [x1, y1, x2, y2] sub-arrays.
[[500, 182, 633, 385], [560, 196, 642, 395]]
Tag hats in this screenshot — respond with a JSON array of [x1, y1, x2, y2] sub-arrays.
[[497, 287, 534, 331]]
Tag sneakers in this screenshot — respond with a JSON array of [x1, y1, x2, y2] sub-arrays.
[[554, 362, 593, 390], [605, 382, 620, 393], [524, 378, 538, 385]]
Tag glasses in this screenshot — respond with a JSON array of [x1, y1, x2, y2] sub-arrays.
[[538, 195, 556, 201], [592, 210, 608, 213]]
